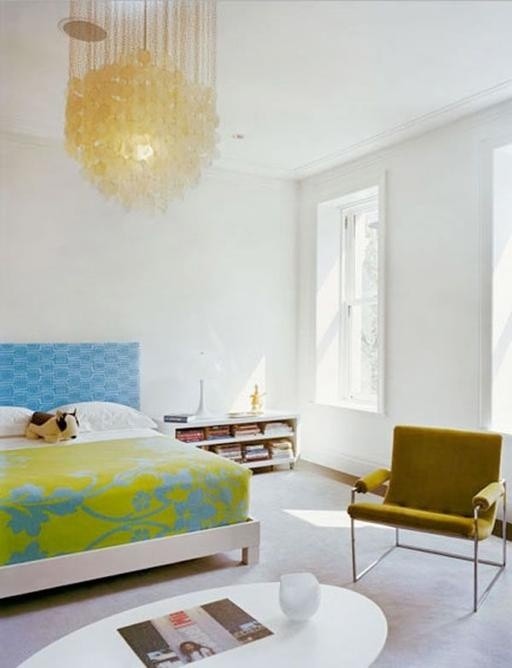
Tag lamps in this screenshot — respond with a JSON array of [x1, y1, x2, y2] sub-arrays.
[[64, 0, 221, 215]]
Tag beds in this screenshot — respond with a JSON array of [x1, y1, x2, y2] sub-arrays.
[[0, 340, 261, 598]]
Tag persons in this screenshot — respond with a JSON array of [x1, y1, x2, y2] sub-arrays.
[[180, 641, 214, 664]]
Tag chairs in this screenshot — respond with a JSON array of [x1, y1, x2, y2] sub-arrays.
[[347, 425, 507, 614]]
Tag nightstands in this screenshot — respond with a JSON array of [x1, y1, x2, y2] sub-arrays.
[[151, 411, 300, 472]]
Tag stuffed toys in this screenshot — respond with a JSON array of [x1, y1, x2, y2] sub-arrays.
[[25, 408, 80, 443]]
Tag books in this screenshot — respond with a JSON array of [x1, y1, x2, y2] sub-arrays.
[[175, 422, 293, 464], [163, 414, 197, 423]]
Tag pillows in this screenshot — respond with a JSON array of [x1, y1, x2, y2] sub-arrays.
[[0, 400, 158, 437]]
